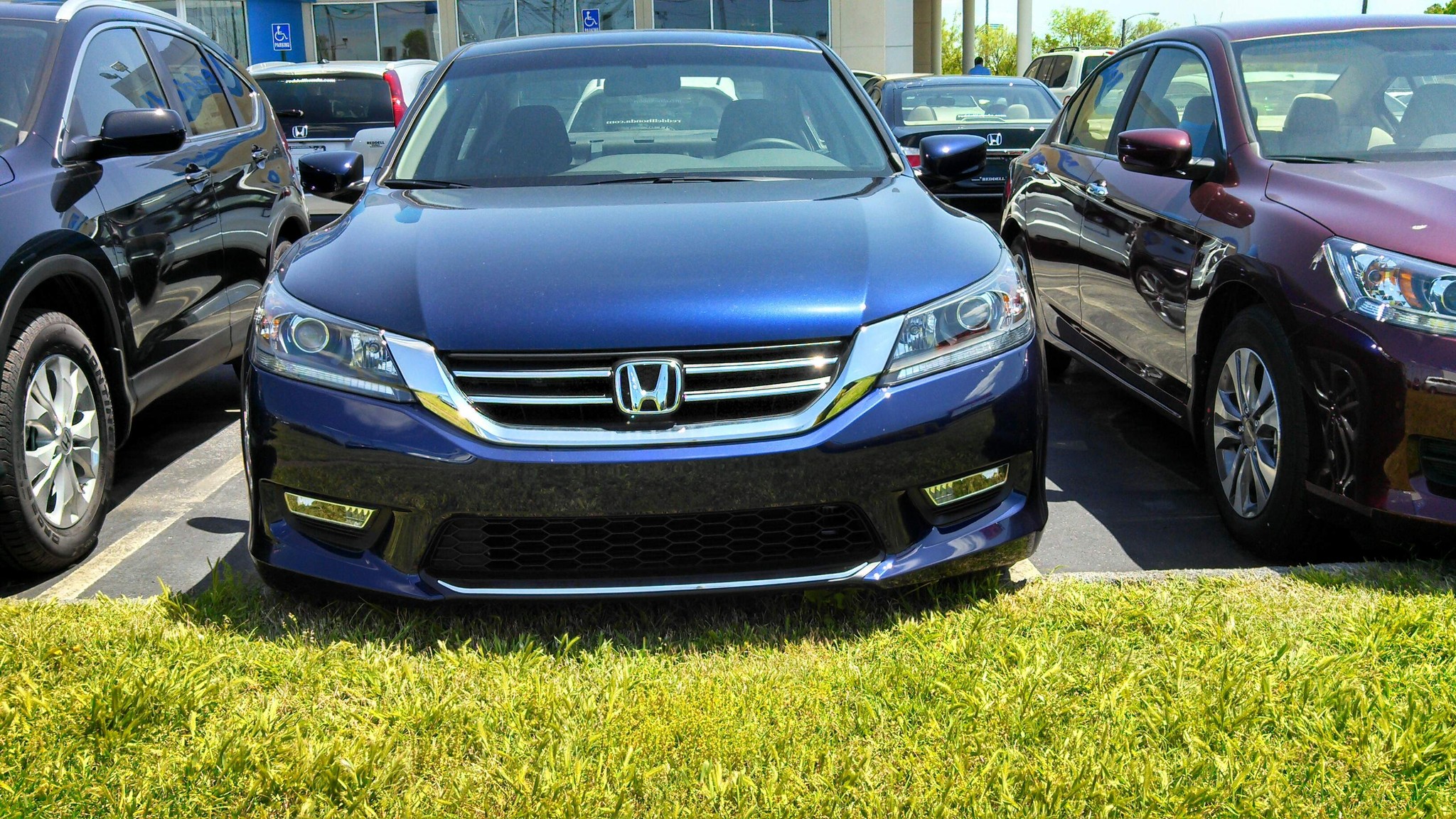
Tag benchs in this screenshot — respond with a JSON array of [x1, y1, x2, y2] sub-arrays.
[[1072, 91, 1396, 155], [566, 135, 713, 163]]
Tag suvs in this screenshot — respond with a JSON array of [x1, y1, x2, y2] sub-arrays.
[[1023, 46, 1140, 119], [245, 58, 489, 239]]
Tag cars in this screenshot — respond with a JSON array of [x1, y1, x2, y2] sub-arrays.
[[997, 12, 1456, 564], [0, 0, 311, 574], [997, 165, 1455, 489], [866, 76, 1064, 234], [862, 73, 985, 122], [1164, 70, 1411, 152], [83, 150, 307, 288], [563, 76, 739, 134], [241, 28, 1051, 602]]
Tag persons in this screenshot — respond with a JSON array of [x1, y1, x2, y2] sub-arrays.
[[968, 57, 992, 75]]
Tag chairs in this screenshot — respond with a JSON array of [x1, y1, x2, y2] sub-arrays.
[[1366, 82, 1456, 152], [495, 104, 580, 176], [984, 105, 1007, 115], [711, 98, 792, 159], [907, 106, 935, 122], [1179, 97, 1224, 155], [1006, 104, 1030, 120]]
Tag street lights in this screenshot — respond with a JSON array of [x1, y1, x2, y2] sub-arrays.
[[1122, 12, 1160, 47]]
